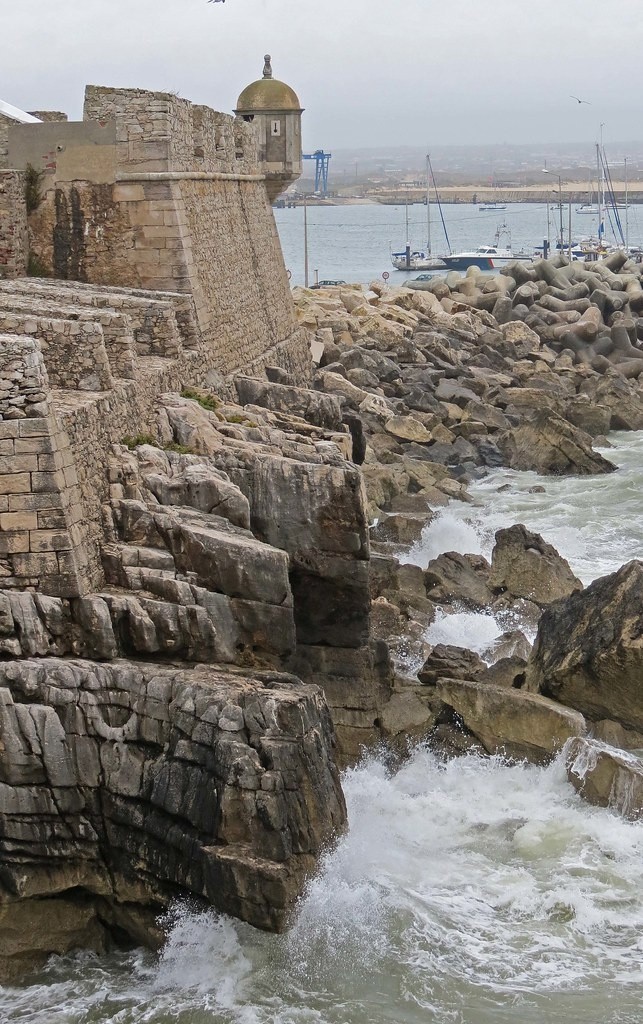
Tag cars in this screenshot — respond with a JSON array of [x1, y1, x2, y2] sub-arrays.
[[318, 279, 348, 285], [409, 273, 445, 290]]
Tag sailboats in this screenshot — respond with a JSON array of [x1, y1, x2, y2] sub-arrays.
[[425, 154, 542, 272], [553, 143, 643, 262], [390, 154, 452, 271]]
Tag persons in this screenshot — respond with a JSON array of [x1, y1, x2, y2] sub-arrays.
[[596, 247, 608, 260]]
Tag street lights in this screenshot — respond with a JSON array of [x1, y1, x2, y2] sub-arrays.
[[541, 168, 564, 255]]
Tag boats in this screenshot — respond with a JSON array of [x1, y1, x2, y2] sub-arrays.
[[479, 202, 507, 209], [575, 205, 606, 214], [603, 201, 631, 209], [550, 204, 568, 210]]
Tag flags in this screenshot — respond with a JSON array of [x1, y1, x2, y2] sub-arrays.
[[598, 223, 604, 238]]
